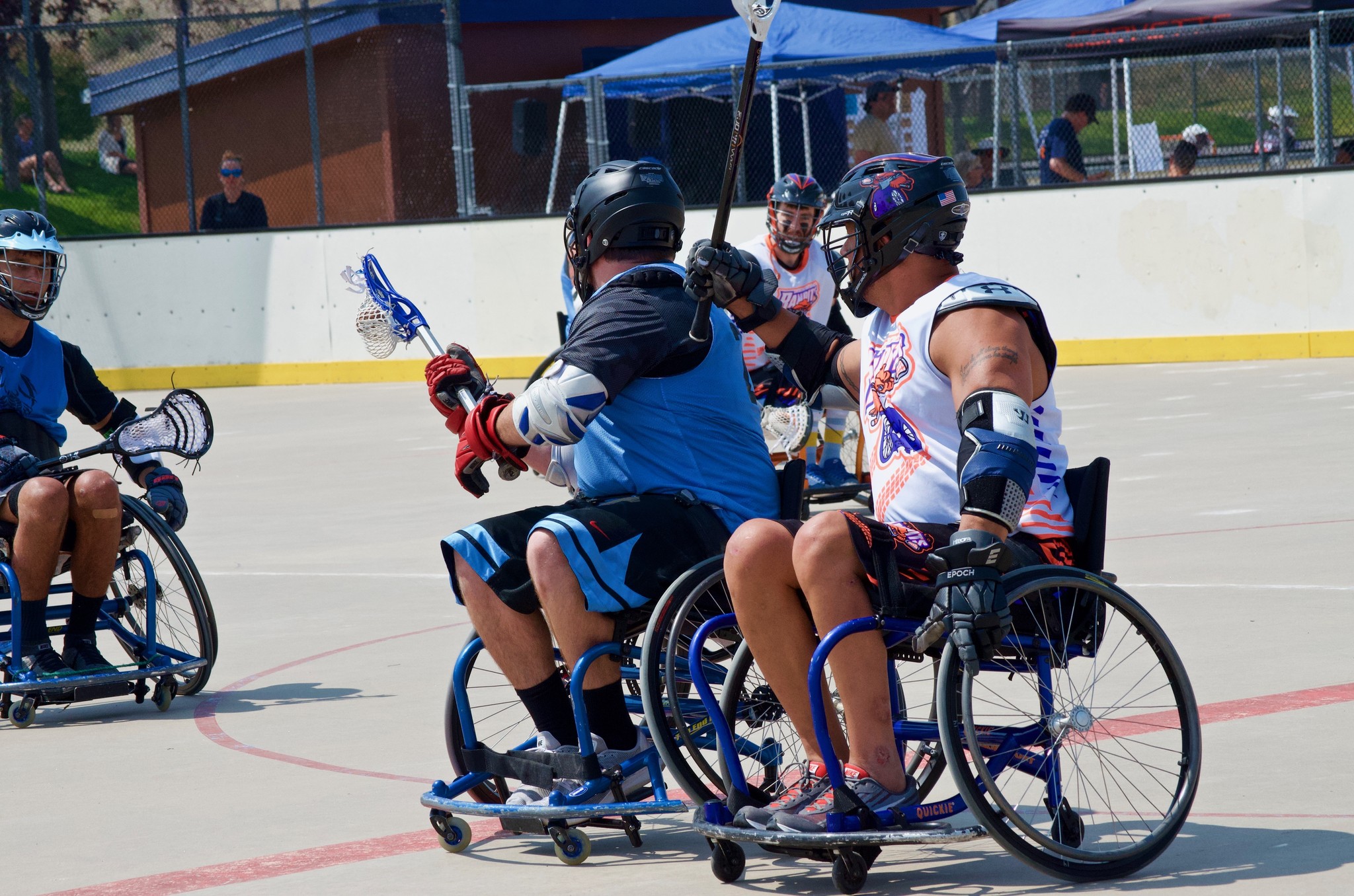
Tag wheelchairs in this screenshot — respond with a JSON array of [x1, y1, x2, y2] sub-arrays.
[[689, 470, 1203, 895], [419, 452, 907, 867], [1, 464, 218, 729]]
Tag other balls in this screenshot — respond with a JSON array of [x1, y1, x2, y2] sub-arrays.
[[356, 306, 386, 339]]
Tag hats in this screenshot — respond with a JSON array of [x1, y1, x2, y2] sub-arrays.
[[952, 152, 981, 187], [1066, 92, 1100, 124], [865, 82, 900, 100], [970, 138, 1010, 159]]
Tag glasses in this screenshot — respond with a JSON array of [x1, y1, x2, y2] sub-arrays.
[[220, 168, 242, 178]]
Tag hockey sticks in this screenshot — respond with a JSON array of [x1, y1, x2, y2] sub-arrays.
[[356, 253, 521, 481], [32, 388, 214, 470], [690, 0, 782, 343], [760, 401, 813, 453]]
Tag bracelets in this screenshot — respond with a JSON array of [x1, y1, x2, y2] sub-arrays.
[[1082, 176, 1087, 183]]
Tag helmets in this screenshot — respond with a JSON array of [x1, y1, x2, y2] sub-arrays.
[[1266, 104, 1299, 136], [1182, 123, 1210, 147], [0, 208, 67, 321], [817, 151, 970, 318], [765, 172, 828, 254], [562, 158, 686, 305]]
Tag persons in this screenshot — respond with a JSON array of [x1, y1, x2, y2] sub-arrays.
[[1040, 93, 1114, 185], [854, 77, 902, 165], [96, 113, 137, 174], [0, 205, 188, 695], [423, 137, 1072, 843], [1252, 104, 1300, 153], [11, 116, 75, 195], [968, 137, 1028, 188], [1332, 138, 1354, 165], [198, 149, 269, 233], [1180, 123, 1216, 157], [1169, 139, 1198, 177], [947, 152, 986, 194]]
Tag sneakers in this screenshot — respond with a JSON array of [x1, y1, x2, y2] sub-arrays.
[[61, 630, 120, 677], [505, 731, 580, 805], [10, 616, 82, 694], [761, 764, 921, 833], [529, 724, 666, 826], [733, 760, 845, 831]]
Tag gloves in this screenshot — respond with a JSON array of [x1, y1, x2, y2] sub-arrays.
[[912, 528, 1012, 676], [424, 343, 499, 439], [144, 466, 188, 532], [681, 238, 778, 309], [0, 437, 41, 492], [453, 391, 530, 499]]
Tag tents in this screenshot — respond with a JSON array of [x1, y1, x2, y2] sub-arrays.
[[544, 0, 1354, 216]]
